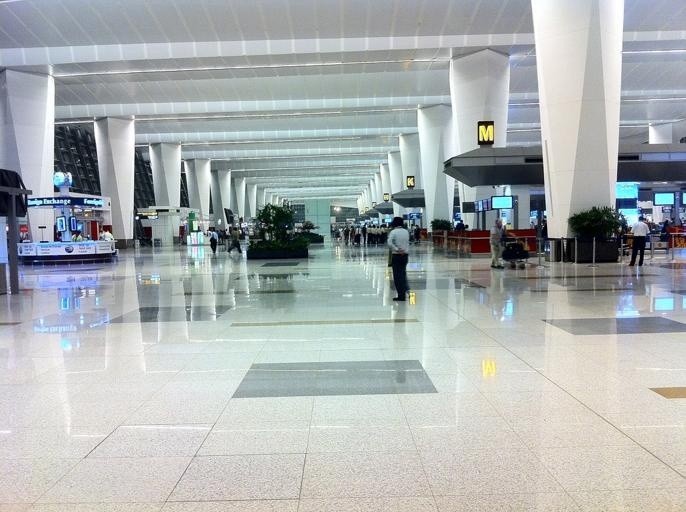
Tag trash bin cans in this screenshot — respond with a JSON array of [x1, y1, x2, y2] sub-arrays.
[[544, 237, 575, 263], [135, 240, 140, 249]]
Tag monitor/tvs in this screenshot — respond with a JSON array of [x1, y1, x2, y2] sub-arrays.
[[491, 195, 514, 211], [653, 193, 675, 207], [680, 191, 686, 207]]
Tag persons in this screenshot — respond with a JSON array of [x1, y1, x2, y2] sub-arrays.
[[57, 227, 115, 242], [206, 225, 248, 253], [387, 216, 410, 300], [455, 219, 465, 230], [415, 225, 421, 243], [542, 221, 547, 251], [498, 220, 506, 238], [331, 222, 393, 247], [615, 211, 686, 249], [411, 224, 416, 242], [530, 221, 535, 229], [489, 220, 504, 268], [629, 216, 651, 266], [19, 230, 28, 242]]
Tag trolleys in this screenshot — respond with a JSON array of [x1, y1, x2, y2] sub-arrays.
[[501, 237, 530, 271]]
